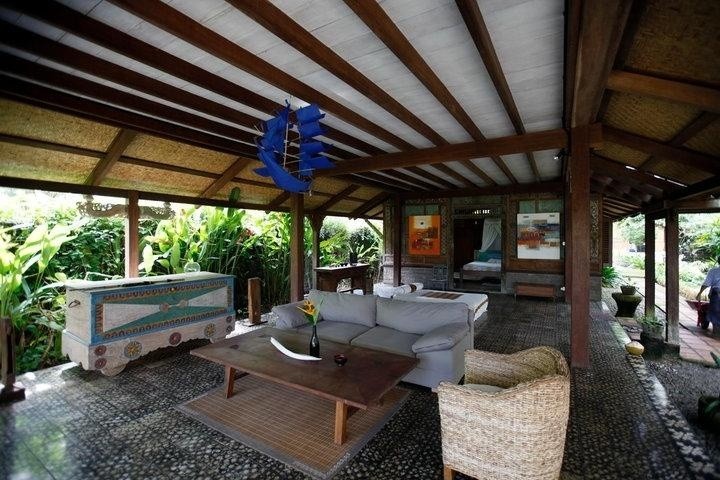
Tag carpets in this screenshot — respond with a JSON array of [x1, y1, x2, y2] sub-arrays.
[[173, 374, 412, 480]]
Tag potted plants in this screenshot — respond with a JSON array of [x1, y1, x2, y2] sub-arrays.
[[639, 308, 664, 351], [602, 266, 616, 283]]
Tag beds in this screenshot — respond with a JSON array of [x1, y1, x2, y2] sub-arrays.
[[462, 250, 502, 280], [394, 282, 487, 324]]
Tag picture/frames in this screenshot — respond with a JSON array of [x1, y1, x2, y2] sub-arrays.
[[517, 213, 560, 259], [407, 214, 440, 257]]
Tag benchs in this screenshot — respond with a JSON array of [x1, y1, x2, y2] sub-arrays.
[[512, 282, 556, 302]]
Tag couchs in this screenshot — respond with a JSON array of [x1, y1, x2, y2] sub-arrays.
[[271, 290, 474, 389]]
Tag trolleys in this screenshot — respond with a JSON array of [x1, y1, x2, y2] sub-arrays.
[[687, 298, 715, 330]]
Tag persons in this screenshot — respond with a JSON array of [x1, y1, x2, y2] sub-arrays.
[[695, 256, 720, 336]]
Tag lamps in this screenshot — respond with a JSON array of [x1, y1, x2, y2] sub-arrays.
[[552, 147, 569, 161]]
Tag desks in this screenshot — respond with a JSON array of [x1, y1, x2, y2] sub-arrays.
[[62, 272, 236, 376], [314, 263, 371, 295]]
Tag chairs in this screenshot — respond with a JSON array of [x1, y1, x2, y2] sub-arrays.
[[429, 265, 449, 291], [436, 346, 571, 480]]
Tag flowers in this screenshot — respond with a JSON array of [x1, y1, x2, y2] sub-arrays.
[[296, 297, 322, 326]]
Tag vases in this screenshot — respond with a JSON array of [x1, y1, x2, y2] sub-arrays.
[[310, 326, 320, 358]]
